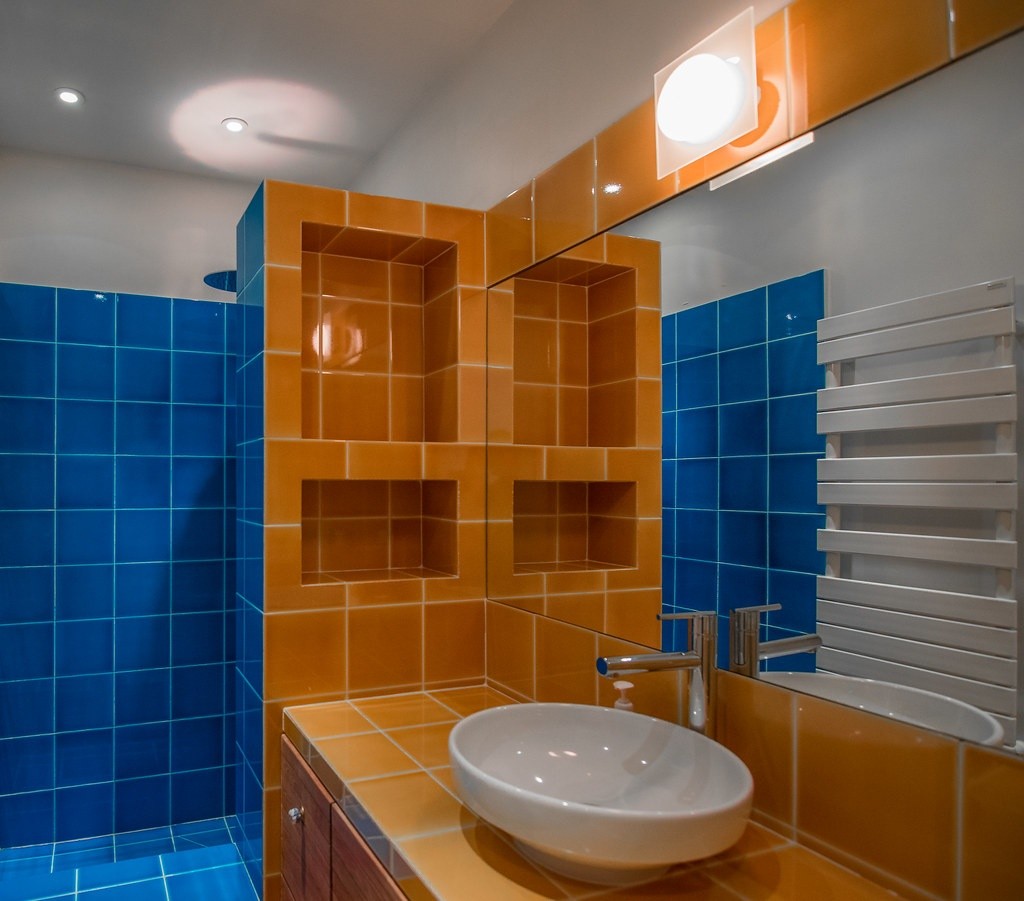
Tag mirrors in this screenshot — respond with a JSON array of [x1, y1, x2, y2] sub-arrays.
[[484, 28, 1024, 762]]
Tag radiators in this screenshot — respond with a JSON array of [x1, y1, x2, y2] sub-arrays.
[[813, 276, 1019, 747]]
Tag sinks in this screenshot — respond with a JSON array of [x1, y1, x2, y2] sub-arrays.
[[448, 704, 753, 872], [758, 673, 1005, 749]]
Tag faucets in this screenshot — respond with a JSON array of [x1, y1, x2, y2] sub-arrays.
[[596, 611, 718, 739], [731, 604, 823, 681]]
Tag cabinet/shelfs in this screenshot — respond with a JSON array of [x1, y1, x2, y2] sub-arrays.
[[277, 734, 408, 900]]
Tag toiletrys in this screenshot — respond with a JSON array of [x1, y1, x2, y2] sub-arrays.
[[612, 680, 635, 712]]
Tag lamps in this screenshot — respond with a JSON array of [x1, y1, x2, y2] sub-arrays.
[[652, 4, 760, 182]]
